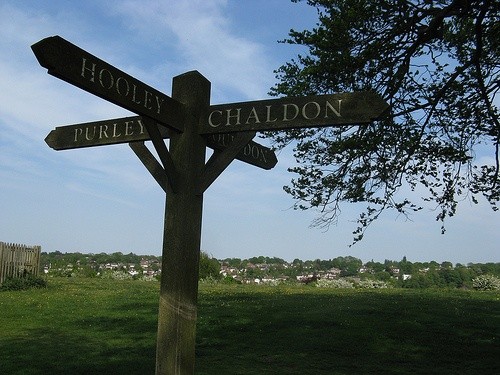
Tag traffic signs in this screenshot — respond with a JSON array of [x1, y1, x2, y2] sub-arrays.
[[29, 34, 393, 170]]
